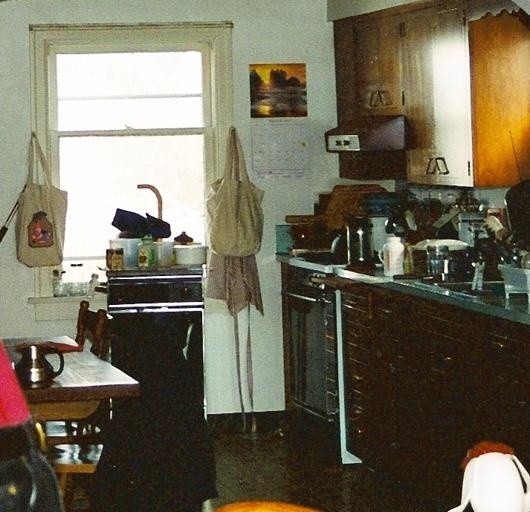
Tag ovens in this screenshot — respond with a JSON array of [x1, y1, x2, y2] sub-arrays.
[[101, 278, 207, 425], [278, 280, 333, 425]]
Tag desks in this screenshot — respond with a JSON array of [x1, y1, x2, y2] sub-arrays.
[[0, 335, 141, 511]]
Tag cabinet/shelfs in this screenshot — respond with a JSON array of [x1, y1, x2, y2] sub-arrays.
[[332, 0, 530, 188]]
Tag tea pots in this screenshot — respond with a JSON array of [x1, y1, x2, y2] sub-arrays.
[[11, 344, 65, 389]]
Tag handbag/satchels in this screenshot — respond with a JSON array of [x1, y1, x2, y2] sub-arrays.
[[205, 125, 264, 258], [17, 132, 67, 268]]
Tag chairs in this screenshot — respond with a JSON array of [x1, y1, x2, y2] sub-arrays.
[[33, 300, 114, 511]]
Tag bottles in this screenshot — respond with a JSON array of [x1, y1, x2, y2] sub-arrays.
[[427, 245, 450, 277], [105, 248, 125, 272], [377, 235, 404, 278]]
